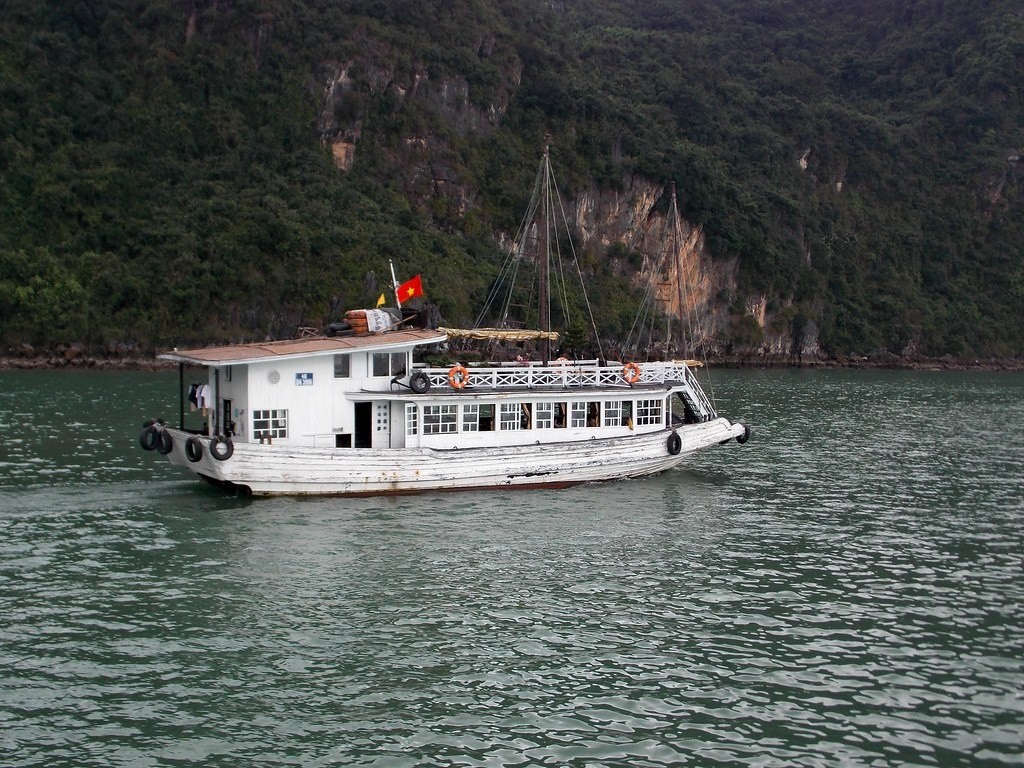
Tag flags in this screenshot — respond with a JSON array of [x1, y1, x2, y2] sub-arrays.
[[397, 275, 422, 303]]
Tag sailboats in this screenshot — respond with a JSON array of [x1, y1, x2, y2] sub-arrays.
[[141, 133, 751, 499]]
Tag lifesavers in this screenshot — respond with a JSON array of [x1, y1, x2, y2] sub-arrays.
[[210, 436, 234, 461], [409, 372, 431, 394], [326, 322, 355, 337], [138, 418, 173, 456], [554, 356, 571, 375], [666, 433, 682, 455], [735, 424, 751, 444], [448, 366, 469, 389], [623, 361, 640, 384], [184, 436, 202, 462], [718, 438, 732, 445]]
